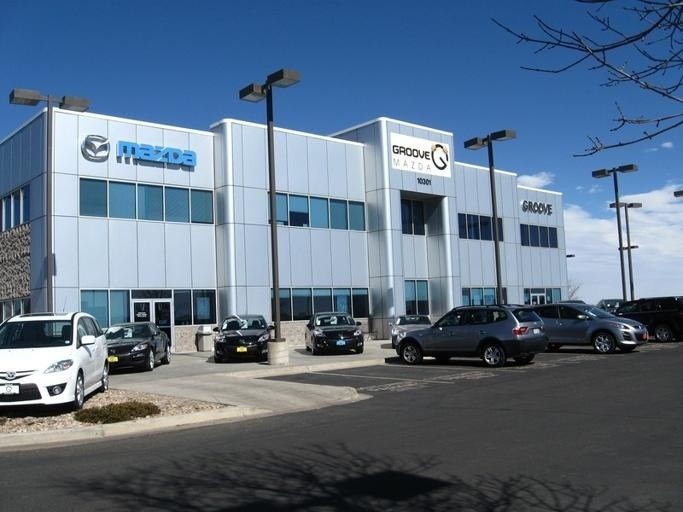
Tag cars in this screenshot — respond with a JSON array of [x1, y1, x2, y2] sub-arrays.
[[0, 311, 109, 413], [388, 296, 682, 368], [103, 321, 170, 376], [304, 312, 363, 355], [212, 315, 276, 363]]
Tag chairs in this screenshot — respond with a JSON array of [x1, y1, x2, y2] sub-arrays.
[[227, 321, 239, 330], [472, 312, 483, 322], [62, 325, 73, 342], [252, 320, 259, 329], [19, 323, 44, 344]]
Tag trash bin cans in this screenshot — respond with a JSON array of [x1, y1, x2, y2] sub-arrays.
[[197, 325, 213, 352]]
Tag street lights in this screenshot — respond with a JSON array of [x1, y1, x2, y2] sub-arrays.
[[591, 164, 637, 305], [463, 129, 516, 320], [8, 87, 89, 339], [238, 70, 300, 344], [608, 202, 641, 304]]
